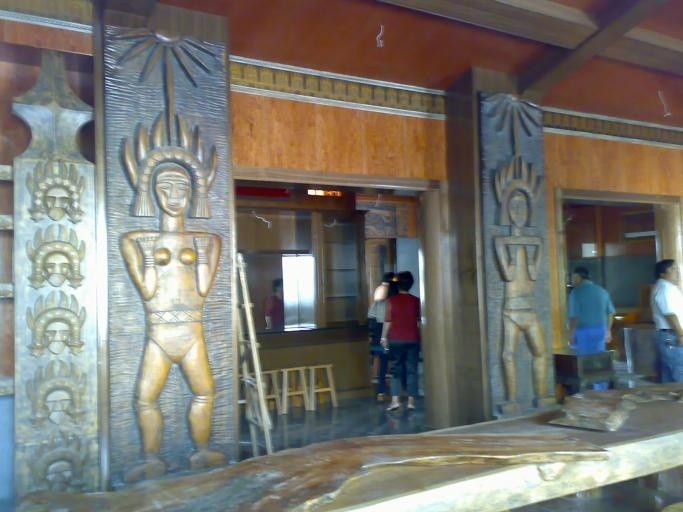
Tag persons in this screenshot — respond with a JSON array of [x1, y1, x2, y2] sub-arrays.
[[648, 258, 682, 384], [365, 271, 392, 403], [45, 320, 71, 354], [43, 461, 76, 493], [379, 271, 420, 413], [43, 187, 74, 222], [117, 165, 231, 478], [262, 277, 284, 330], [492, 189, 559, 412], [560, 266, 616, 393], [43, 389, 72, 426], [46, 254, 70, 286]]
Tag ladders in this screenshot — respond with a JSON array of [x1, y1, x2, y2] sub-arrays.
[[236, 252, 272, 459]]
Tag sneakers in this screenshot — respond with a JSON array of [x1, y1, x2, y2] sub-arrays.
[[385, 404, 399, 411], [407, 405, 414, 414], [377, 393, 391, 402], [385, 375, 391, 383], [370, 376, 379, 384]]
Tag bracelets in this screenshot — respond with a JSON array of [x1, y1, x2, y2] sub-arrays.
[[380, 338, 387, 341]]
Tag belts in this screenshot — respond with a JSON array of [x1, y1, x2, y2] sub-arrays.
[[655, 328, 674, 332]]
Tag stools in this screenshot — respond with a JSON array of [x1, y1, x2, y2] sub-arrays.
[[239, 363, 338, 415]]
